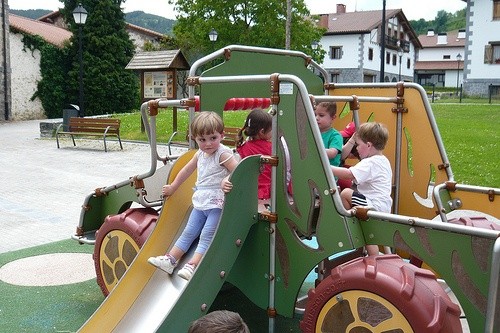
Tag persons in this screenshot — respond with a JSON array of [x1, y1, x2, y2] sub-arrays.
[[314, 101, 344, 183], [147, 110, 238, 280], [233, 108, 272, 201], [330, 122, 393, 255]]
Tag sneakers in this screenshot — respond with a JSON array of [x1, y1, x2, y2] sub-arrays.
[[147, 256, 176, 274], [177, 264, 193, 280]]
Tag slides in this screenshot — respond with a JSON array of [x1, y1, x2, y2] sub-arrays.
[[74, 149, 259, 333]]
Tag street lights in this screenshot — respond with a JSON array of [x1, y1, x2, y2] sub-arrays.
[[311, 40, 318, 73], [72, 3, 88, 137], [396, 46, 404, 82], [455, 53, 463, 96], [208, 27, 219, 67]]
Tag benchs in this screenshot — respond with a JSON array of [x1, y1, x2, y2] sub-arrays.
[[55, 117, 123, 152], [186, 123, 243, 149]]
